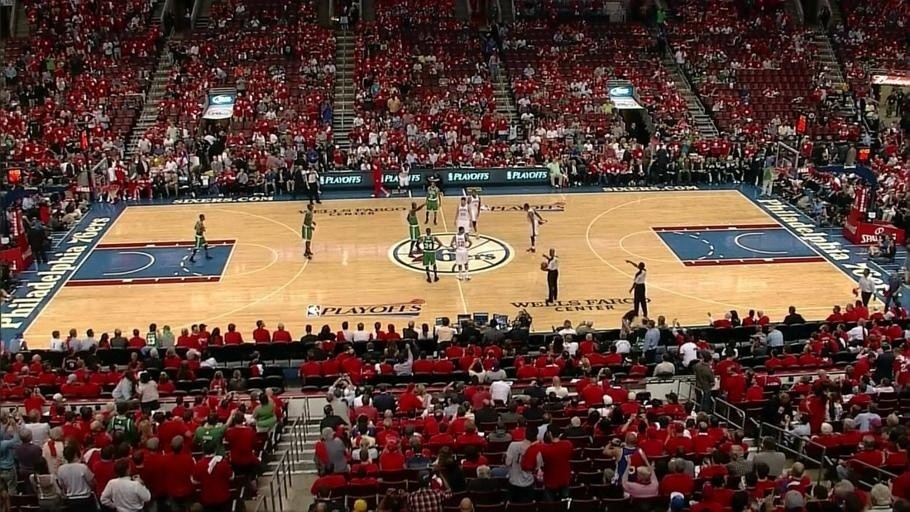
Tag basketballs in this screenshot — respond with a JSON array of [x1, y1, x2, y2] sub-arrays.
[[540, 262, 549, 270]]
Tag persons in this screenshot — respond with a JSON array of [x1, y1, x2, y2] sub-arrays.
[[1, 3, 910, 512]]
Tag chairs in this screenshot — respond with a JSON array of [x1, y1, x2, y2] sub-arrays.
[[285, 320, 910, 512], [0, 343, 284, 512], [4, 36, 27, 64], [417, 63, 476, 91], [505, 21, 656, 130], [249, 0, 279, 7], [864, 16, 895, 41], [715, 49, 843, 136], [229, 57, 301, 142], [96, 24, 149, 137]]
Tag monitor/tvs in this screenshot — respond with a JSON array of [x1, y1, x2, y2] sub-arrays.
[[458, 314, 471, 326], [474, 313, 489, 325], [493, 314, 507, 326], [436, 317, 443, 325]]
